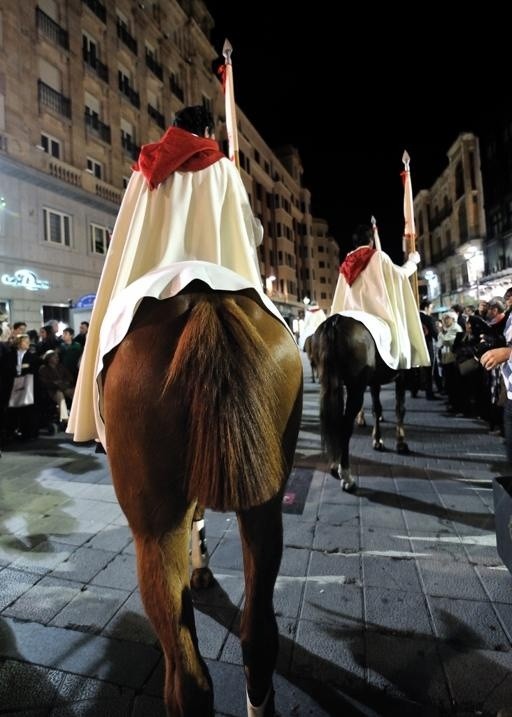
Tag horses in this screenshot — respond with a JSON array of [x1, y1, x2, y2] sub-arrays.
[[305, 314, 429, 493], [102, 279, 302, 717]]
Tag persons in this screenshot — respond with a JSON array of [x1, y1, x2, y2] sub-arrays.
[[0, 319, 89, 446], [412, 291, 512, 463], [65, 107, 297, 455], [330, 225, 433, 371], [300, 301, 327, 349]]
